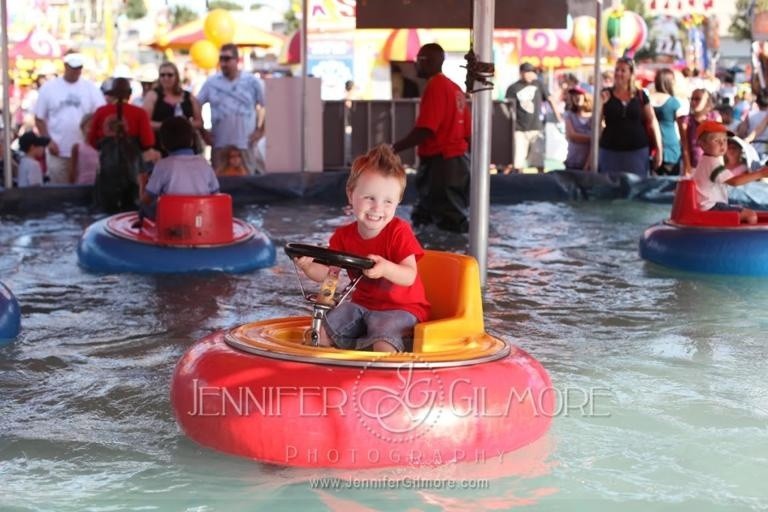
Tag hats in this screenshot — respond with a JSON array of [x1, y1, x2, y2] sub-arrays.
[[520, 63, 533, 71], [64, 52, 83, 69], [568, 87, 584, 94], [695, 120, 734, 141], [112, 63, 132, 80], [18, 131, 50, 149]]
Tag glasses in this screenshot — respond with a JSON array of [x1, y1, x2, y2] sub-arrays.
[[617, 57, 631, 65], [219, 55, 235, 60], [159, 72, 174, 77]]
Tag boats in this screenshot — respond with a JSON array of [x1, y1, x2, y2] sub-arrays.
[[634, 176, 767, 279], [171, 243, 557, 467], [76, 195, 275, 278]]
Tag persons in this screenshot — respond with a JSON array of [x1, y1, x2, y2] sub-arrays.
[[495, 40, 767, 225], [389, 41, 472, 235], [291, 141, 429, 355], [1, 39, 269, 228], [341, 80, 365, 129]]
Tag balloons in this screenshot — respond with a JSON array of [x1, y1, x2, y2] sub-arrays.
[[203, 8, 237, 49]]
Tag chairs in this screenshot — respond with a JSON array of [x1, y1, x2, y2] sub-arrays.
[[412, 250, 485, 354], [672, 179, 741, 226], [141, 193, 235, 246]]
